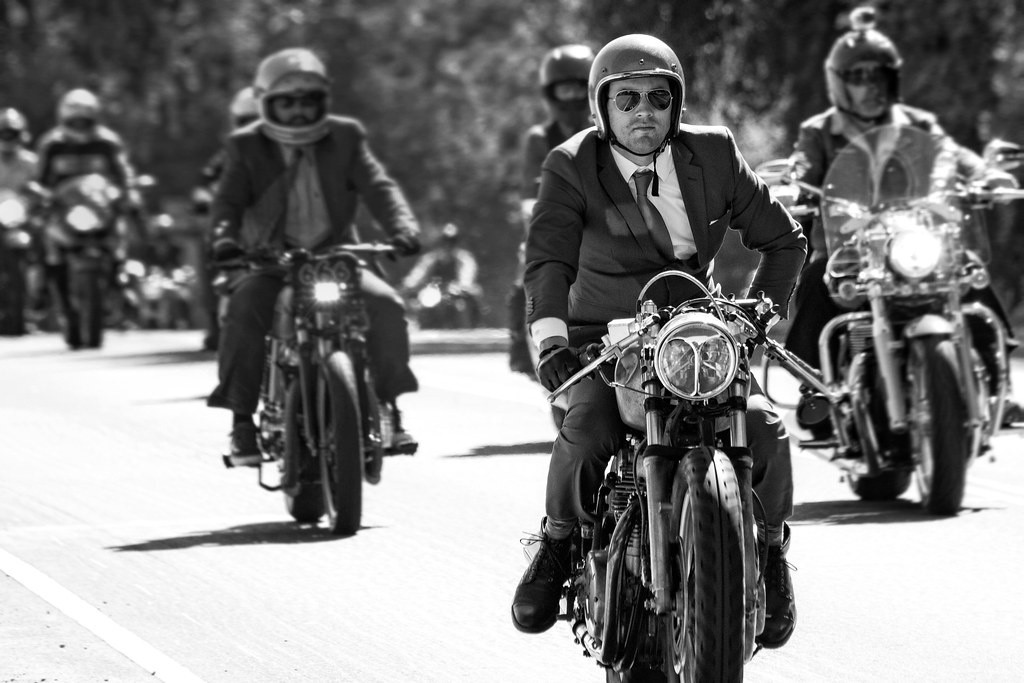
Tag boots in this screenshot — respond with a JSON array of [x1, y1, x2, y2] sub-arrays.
[[512, 517, 578, 634], [752, 523, 796, 648]]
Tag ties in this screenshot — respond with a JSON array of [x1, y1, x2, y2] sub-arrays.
[[633, 171, 674, 259]]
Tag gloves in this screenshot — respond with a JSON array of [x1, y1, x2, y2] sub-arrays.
[[536, 345, 596, 392], [384, 231, 421, 262], [217, 246, 251, 270]]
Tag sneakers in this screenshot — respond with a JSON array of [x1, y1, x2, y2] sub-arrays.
[[232, 422, 263, 465], [990, 398, 1024, 429], [379, 405, 418, 452]]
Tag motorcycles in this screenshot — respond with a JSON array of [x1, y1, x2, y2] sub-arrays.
[[751, 137, 1024, 519], [546, 269, 870, 682], [403, 277, 483, 329], [0, 176, 198, 349], [221, 240, 407, 535]]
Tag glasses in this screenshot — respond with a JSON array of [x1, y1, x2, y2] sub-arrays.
[[606, 89, 673, 113], [844, 67, 889, 83]]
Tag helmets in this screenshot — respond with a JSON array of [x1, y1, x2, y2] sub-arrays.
[[231, 87, 261, 129], [57, 88, 100, 129], [824, 30, 903, 109], [538, 44, 595, 122], [589, 34, 686, 139], [0, 107, 32, 153], [253, 48, 331, 144]]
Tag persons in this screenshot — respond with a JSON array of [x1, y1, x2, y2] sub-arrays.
[[778, 28, 1024, 441], [512, 33, 809, 650], [516, 44, 596, 222], [0, 44, 480, 463]]
